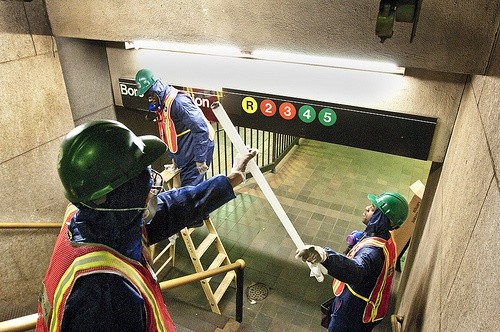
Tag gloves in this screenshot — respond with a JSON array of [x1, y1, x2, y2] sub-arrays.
[[295, 246, 324, 262]]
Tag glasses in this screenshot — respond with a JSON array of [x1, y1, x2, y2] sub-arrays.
[[149, 169, 163, 196]]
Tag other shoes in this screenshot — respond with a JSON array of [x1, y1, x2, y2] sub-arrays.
[[189, 219, 204, 227]]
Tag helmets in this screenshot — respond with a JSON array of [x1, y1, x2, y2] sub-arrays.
[[368, 192, 408, 227], [58, 120, 168, 203], [136, 69, 156, 95]]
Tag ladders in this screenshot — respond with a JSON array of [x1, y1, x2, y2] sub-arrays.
[[149, 168, 236, 315]]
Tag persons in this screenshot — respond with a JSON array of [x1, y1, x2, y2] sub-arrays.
[[36, 120, 257, 332], [295, 192, 409, 332], [136, 68, 216, 228]]
